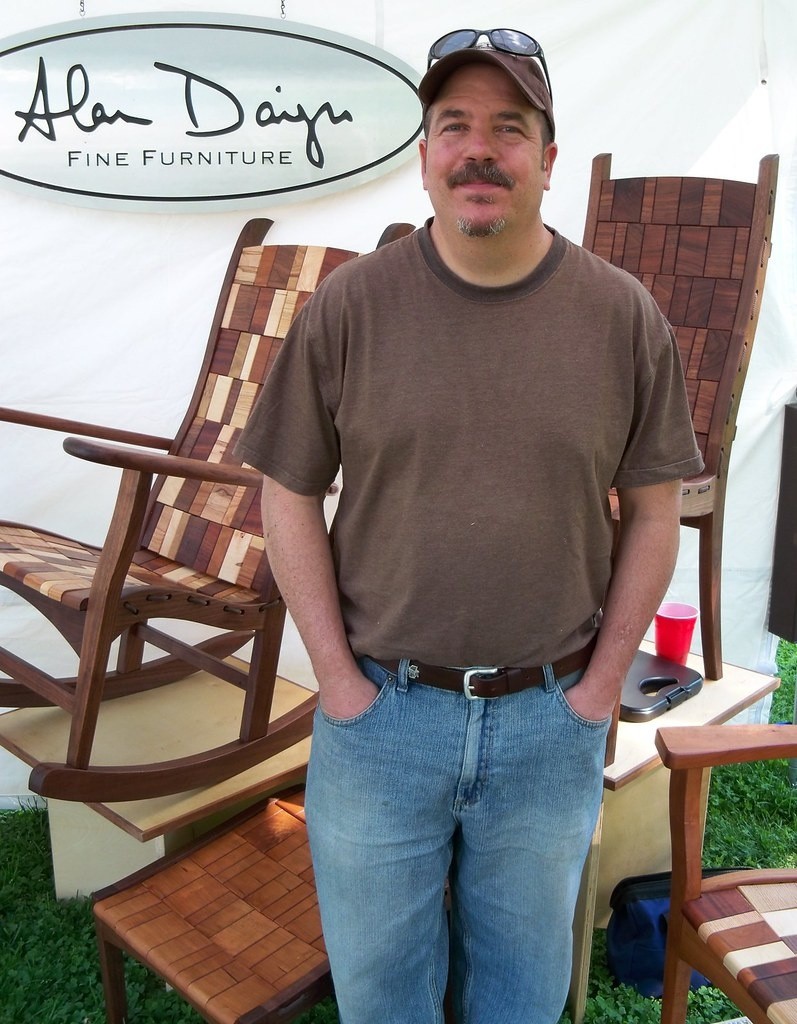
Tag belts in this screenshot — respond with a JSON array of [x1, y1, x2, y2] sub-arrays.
[[368, 634, 596, 701]]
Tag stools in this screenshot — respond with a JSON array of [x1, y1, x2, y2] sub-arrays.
[[90, 782, 451, 1024]]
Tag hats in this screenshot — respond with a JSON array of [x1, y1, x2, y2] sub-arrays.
[[417, 50, 556, 142]]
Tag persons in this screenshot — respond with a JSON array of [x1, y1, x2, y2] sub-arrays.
[[234, 47, 705, 1024]]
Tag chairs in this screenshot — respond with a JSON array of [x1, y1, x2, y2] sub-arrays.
[[582, 153, 780, 767], [0, 217, 418, 803], [654, 724, 797, 1024]]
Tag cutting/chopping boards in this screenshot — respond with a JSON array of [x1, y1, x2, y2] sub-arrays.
[[607, 640, 703, 725]]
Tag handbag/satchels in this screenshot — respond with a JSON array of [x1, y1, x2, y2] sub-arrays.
[[608, 867, 753, 1001]]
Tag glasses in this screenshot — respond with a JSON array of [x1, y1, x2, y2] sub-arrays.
[[427, 28, 553, 105]]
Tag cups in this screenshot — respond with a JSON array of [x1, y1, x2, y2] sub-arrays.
[[654, 602, 699, 665]]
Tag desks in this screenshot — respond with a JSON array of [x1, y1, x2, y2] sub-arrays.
[[0, 641, 780, 1024]]
